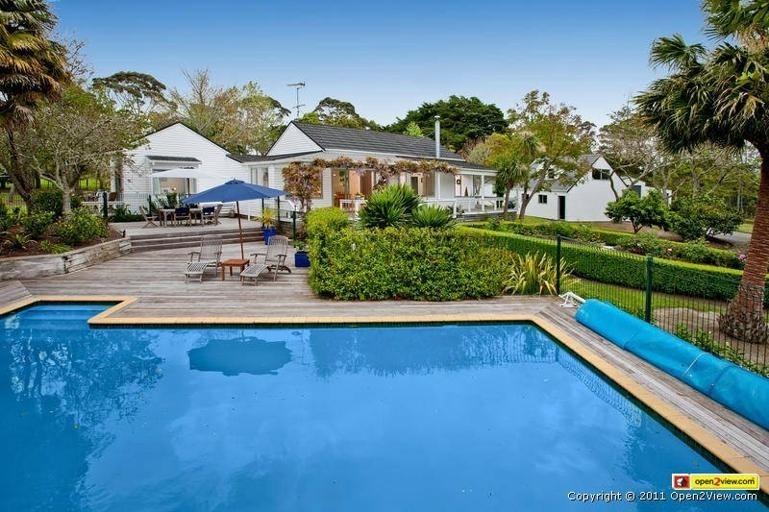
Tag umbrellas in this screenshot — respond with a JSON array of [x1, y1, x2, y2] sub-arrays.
[[180, 177, 290, 259], [187, 327, 293, 376]]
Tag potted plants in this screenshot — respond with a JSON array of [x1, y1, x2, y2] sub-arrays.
[[251, 208, 277, 245], [295, 224, 311, 267]]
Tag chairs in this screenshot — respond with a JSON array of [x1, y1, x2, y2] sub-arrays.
[[137, 204, 223, 229], [185, 234, 290, 287]]
